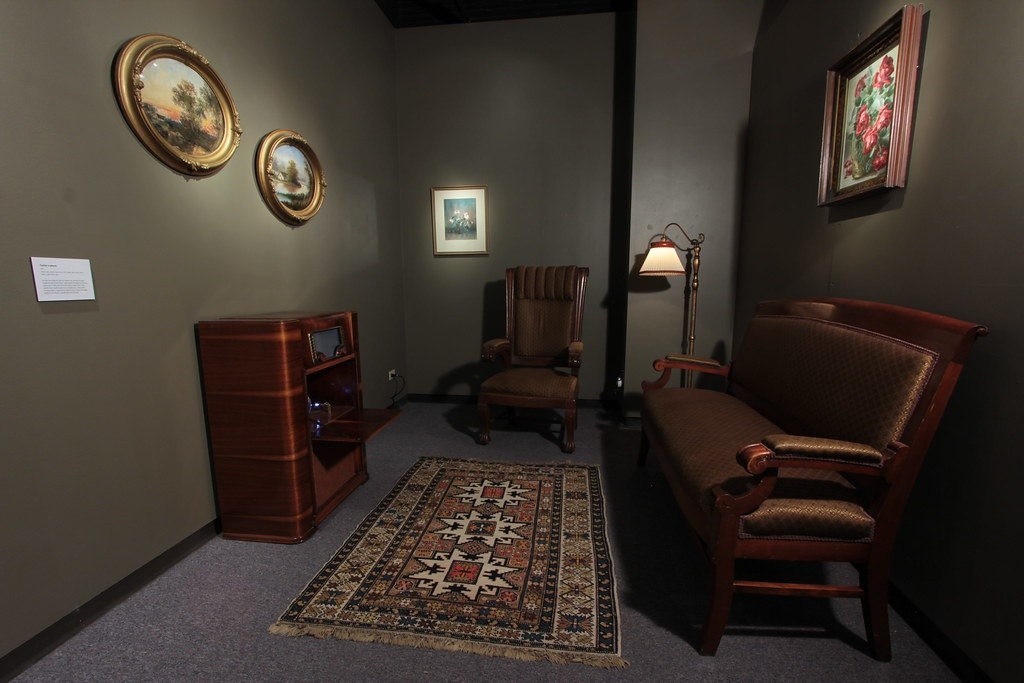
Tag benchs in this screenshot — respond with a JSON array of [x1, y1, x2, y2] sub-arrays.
[[641, 298, 989, 658]]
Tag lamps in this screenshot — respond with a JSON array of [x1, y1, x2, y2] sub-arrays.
[[639, 222, 710, 389]]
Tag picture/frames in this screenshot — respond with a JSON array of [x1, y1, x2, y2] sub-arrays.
[[429, 183, 490, 257], [254, 130, 327, 227], [816, 5, 925, 209], [114, 35, 237, 178]]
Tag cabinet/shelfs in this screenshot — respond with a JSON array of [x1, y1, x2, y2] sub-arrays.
[[193, 307, 362, 544]]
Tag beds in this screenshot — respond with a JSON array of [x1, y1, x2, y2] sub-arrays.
[[476, 265, 590, 453]]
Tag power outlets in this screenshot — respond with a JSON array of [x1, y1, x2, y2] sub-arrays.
[[387, 365, 407, 399]]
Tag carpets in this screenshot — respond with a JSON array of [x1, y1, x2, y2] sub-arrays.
[[272, 458, 624, 670]]
